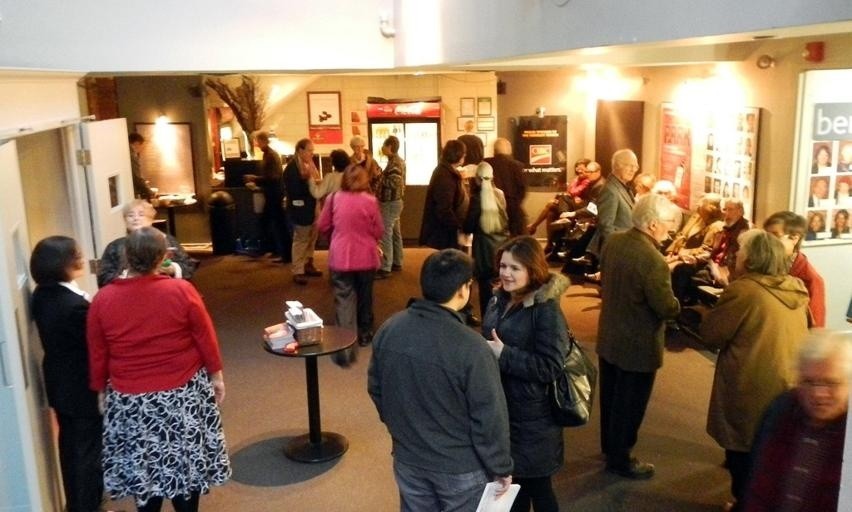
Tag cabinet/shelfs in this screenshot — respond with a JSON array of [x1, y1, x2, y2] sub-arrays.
[[515, 115, 568, 193]]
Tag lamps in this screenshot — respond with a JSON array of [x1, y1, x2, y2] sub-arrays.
[[756, 55, 775, 69]]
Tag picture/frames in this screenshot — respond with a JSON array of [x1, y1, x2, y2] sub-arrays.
[[459, 97, 475, 116], [133, 122, 198, 198], [456, 116, 475, 132], [473, 133, 487, 147], [655, 101, 762, 226], [477, 116, 495, 132], [223, 137, 242, 160], [477, 96, 492, 115], [529, 144, 552, 166], [306, 91, 342, 127]]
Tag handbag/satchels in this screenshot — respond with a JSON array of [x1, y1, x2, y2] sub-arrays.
[[543, 332, 598, 429]]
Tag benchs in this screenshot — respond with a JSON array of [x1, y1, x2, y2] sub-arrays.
[[679, 207, 723, 355]]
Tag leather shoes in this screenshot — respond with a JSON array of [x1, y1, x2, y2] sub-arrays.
[[605, 455, 656, 479], [571, 255, 592, 266], [583, 272, 603, 287]]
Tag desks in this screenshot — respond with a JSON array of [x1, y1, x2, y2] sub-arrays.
[[263, 325, 359, 464], [212, 182, 332, 250], [150, 195, 201, 238]]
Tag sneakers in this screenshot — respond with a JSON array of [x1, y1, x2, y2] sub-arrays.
[[392, 263, 403, 272], [337, 352, 356, 367], [556, 250, 573, 258], [375, 269, 392, 279], [304, 263, 323, 277], [293, 273, 307, 285], [359, 332, 373, 346], [465, 313, 482, 327]]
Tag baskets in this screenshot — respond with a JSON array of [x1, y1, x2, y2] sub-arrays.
[[284, 307, 324, 346]]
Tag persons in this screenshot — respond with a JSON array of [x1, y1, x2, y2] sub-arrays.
[[240, 130, 406, 286], [765, 210, 825, 328], [481, 236, 573, 511], [421, 138, 526, 323], [457, 122, 484, 167], [695, 228, 809, 511], [130, 130, 162, 201], [741, 330, 852, 512], [526, 149, 678, 284], [29, 235, 104, 511], [95, 198, 186, 282], [367, 249, 516, 512], [662, 193, 748, 327], [314, 164, 384, 370], [87, 227, 234, 512], [595, 193, 683, 481], [807, 143, 852, 241]]
